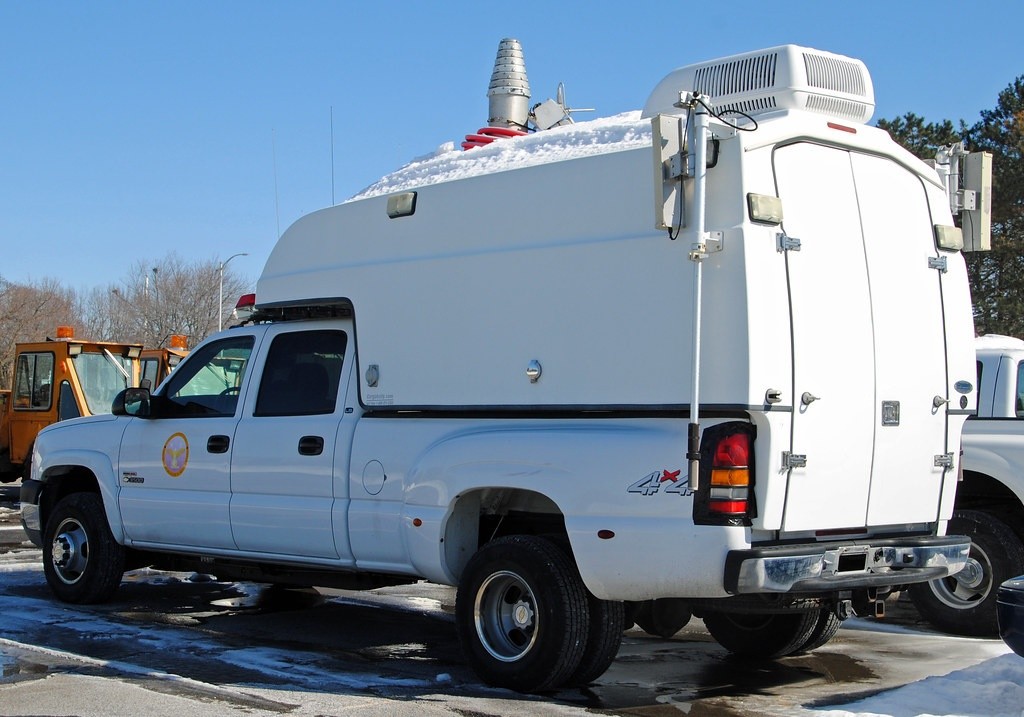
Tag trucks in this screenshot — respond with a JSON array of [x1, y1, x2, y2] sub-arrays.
[[0, 325, 248, 486]]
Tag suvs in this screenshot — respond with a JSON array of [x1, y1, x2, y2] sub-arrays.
[[851, 336, 1024, 640]]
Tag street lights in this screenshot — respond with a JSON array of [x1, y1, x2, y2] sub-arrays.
[[218, 254, 248, 357]]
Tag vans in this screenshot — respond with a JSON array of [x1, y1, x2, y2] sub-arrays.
[[18, 36, 981, 689]]
